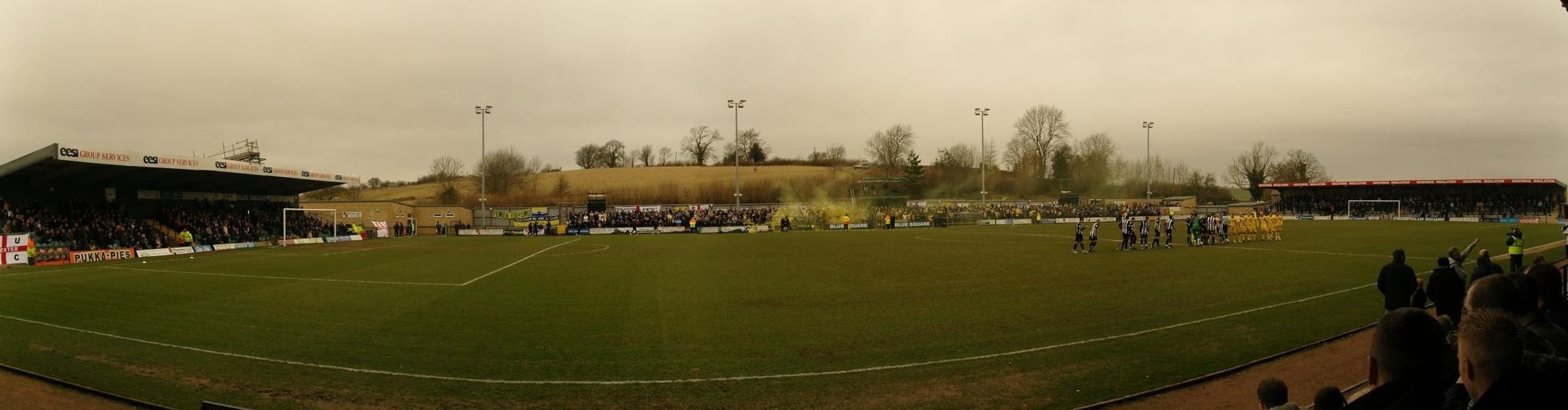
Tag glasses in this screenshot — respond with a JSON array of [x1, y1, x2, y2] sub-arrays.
[[1460, 307, 1471, 314]]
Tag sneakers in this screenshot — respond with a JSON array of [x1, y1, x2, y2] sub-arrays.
[[1071, 236, 1283, 253]]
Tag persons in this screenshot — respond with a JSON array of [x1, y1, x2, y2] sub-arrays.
[[1087, 219, 1102, 253], [442, 226, 445, 236], [1070, 216, 1086, 255], [1183, 208, 1284, 247], [407, 223, 411, 236], [1127, 218, 1137, 249], [1139, 217, 1150, 250], [1505, 226, 1526, 271], [1256, 373, 1302, 409], [1163, 215, 1177, 249], [466, 222, 470, 229], [1150, 217, 1162, 249], [400, 222, 405, 237], [443, 223, 449, 236], [436, 221, 442, 235], [1312, 385, 1349, 409], [532, 221, 539, 236], [527, 221, 533, 236], [546, 219, 551, 234], [1118, 215, 1132, 251], [454, 223, 460, 235], [394, 221, 399, 237], [461, 223, 466, 231]]
